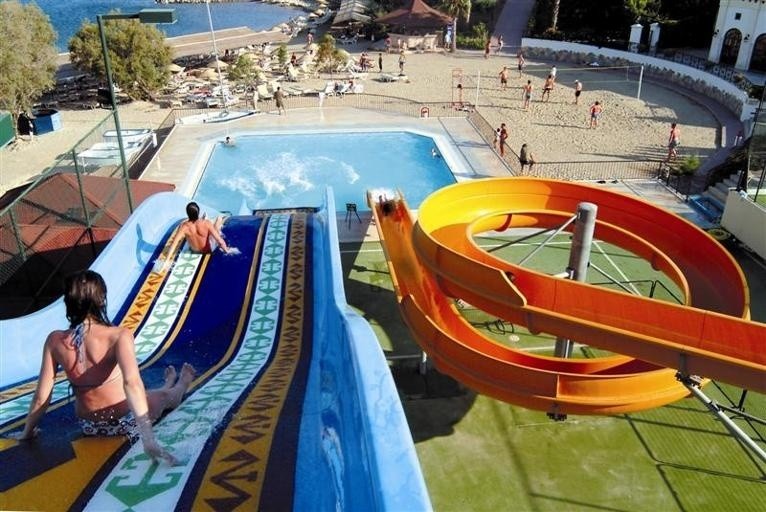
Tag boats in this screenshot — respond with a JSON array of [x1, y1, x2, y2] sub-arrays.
[[76, 128, 153, 168]]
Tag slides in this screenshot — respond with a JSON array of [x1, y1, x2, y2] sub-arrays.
[[366, 178, 765, 416], [0, 186, 434, 511]]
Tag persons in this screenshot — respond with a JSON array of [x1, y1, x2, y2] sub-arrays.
[[379, 194, 392, 215], [161, 31, 407, 112], [273, 87, 287, 116], [224, 137, 233, 145], [432, 148, 438, 158], [6, 268, 202, 466], [156, 201, 241, 264], [664, 122, 680, 163], [483, 34, 604, 170]]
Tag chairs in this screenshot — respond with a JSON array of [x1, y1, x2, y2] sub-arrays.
[[379, 72, 406, 83], [336, 34, 358, 44], [159, 43, 362, 108]]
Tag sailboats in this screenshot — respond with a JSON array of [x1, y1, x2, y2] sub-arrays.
[[175, 0, 255, 129]]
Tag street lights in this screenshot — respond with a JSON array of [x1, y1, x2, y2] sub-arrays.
[[97, 6, 179, 216]]
